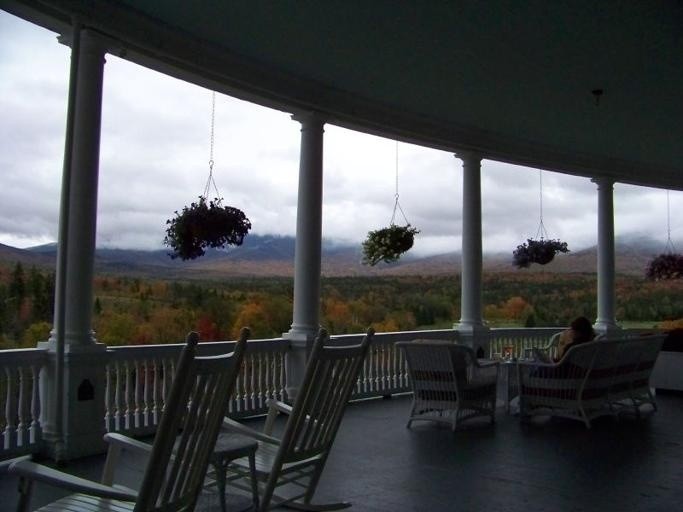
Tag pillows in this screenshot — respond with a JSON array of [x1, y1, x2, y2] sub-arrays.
[[531, 345, 554, 367]]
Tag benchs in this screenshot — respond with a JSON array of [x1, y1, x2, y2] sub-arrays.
[[518, 333, 666, 432]]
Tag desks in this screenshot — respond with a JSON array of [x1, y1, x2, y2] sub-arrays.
[[481, 356, 525, 413], [187, 435, 258, 511]]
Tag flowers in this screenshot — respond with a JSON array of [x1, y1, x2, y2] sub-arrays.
[[642, 253, 683, 285], [361, 224, 421, 268], [512, 235, 570, 270], [163, 193, 252, 262]]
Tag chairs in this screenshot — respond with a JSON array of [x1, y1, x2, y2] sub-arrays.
[[394, 339, 496, 434], [202, 325, 375, 512], [7, 329, 252, 509]]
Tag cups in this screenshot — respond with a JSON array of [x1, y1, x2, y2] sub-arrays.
[[491, 343, 560, 363]]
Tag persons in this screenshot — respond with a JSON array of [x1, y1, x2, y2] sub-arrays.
[[556, 313, 601, 357]]
